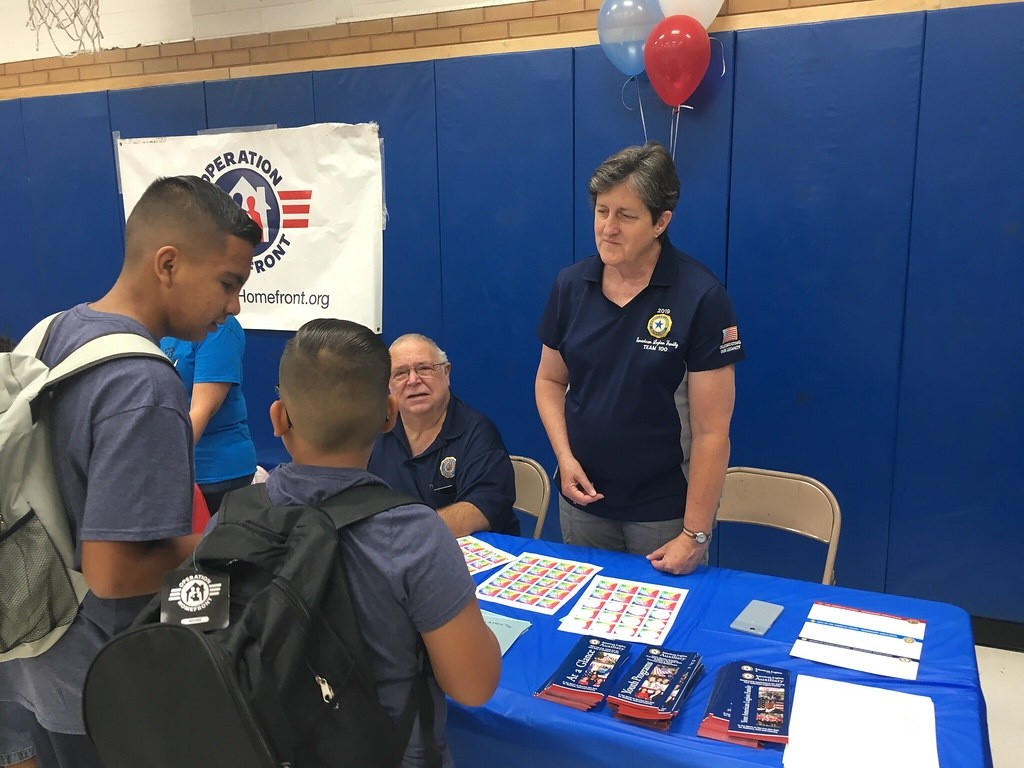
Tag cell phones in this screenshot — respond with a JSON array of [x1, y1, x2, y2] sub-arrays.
[[433, 485, 454, 494], [730, 599, 785, 636]]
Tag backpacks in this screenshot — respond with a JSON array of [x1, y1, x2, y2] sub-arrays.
[[81, 483, 438, 768], [0, 311, 183, 663]]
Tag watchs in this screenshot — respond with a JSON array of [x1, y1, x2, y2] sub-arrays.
[[682, 525, 712, 543]]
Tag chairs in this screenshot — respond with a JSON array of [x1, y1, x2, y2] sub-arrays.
[[717, 466, 841, 585], [511, 455, 550, 539]]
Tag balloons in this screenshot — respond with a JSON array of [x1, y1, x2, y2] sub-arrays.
[[659, 0, 725, 30], [645, 14, 711, 113], [597, 0, 665, 81]]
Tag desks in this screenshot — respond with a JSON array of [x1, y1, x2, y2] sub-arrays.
[[444, 531, 996, 767]]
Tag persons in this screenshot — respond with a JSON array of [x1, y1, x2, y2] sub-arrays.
[[203, 317, 502, 768], [0, 175, 264, 768], [756, 693, 784, 727], [640, 676, 669, 702], [578, 675, 604, 687], [368, 333, 521, 537], [534, 140, 745, 575], [159, 314, 257, 517]]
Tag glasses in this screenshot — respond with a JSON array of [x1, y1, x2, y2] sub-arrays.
[[390, 363, 445, 383]]
